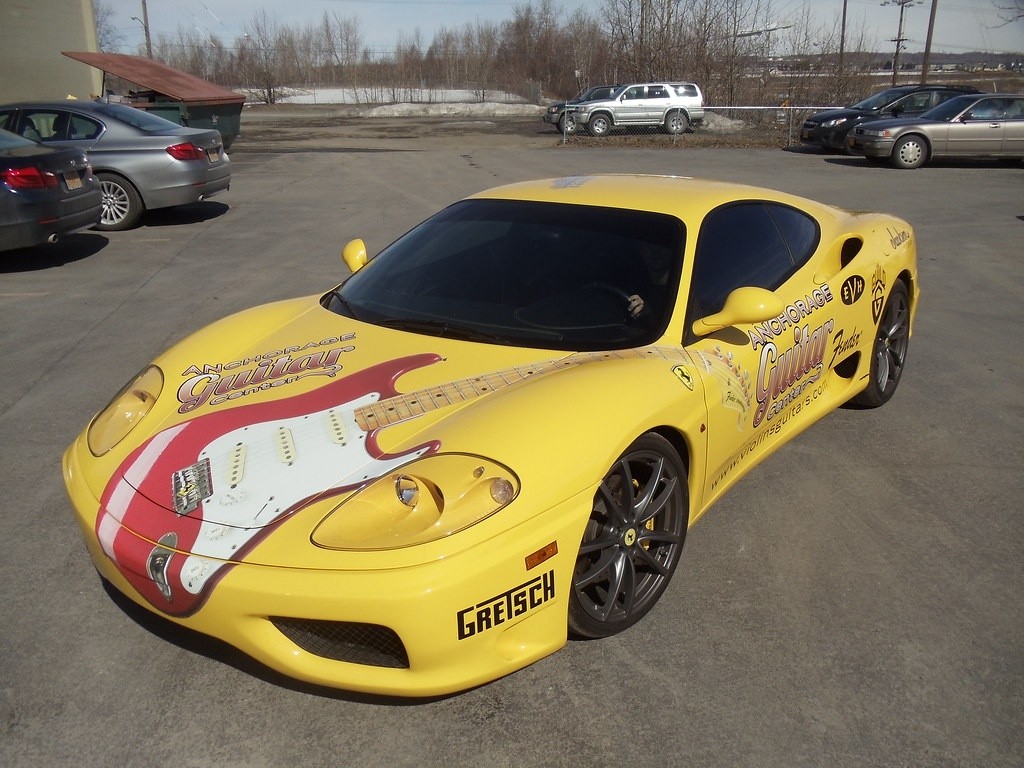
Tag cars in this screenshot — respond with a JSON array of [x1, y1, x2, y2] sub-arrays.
[[0, 101, 234, 232], [1, 128, 103, 251]]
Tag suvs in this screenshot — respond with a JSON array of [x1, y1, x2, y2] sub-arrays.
[[542, 83, 650, 134], [572, 82, 705, 137], [800, 83, 1008, 153], [845, 93, 1024, 169]]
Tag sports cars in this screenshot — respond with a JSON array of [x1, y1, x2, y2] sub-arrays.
[[60, 175, 921, 700]]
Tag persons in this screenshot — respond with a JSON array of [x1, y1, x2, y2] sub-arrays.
[[609, 214, 702, 343]]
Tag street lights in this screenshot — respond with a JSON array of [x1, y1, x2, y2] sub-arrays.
[[132, 16, 152, 60], [880, 0, 924, 87]]
